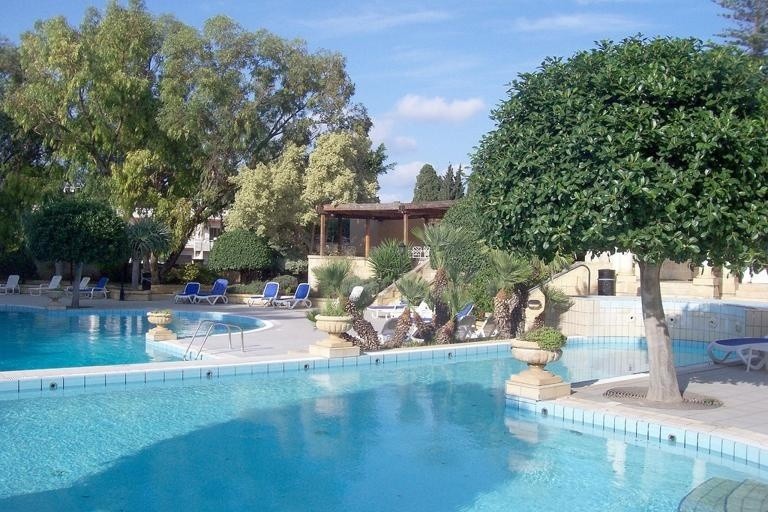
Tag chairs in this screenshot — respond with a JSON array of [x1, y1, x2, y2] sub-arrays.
[[314, 241, 432, 259], [703, 335, 768, 375], [357, 297, 492, 345]]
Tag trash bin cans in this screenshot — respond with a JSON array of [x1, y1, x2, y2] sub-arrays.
[[142, 271, 152, 290], [597, 269, 616, 295]]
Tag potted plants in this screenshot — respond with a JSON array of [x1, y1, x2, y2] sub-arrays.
[[508, 324, 567, 380], [312, 295, 357, 349], [144, 308, 175, 335]]
